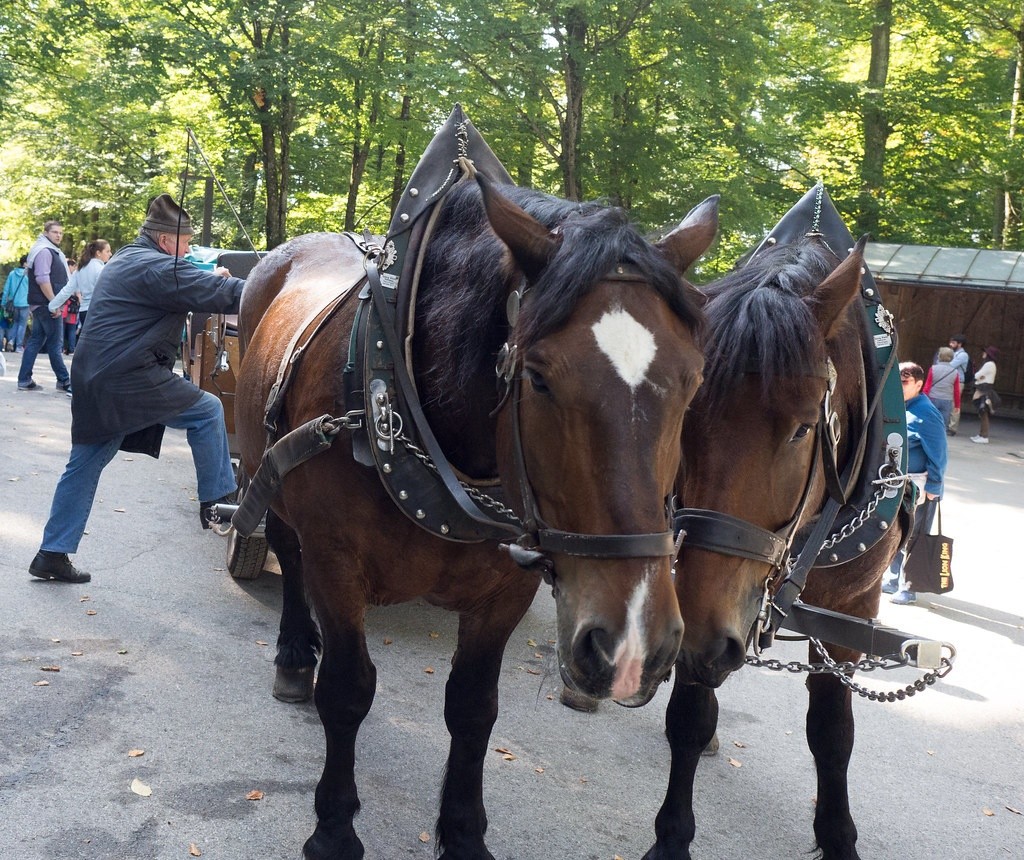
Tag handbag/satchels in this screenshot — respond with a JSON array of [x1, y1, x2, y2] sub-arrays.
[[903, 496, 953, 594], [5, 300, 14, 312]]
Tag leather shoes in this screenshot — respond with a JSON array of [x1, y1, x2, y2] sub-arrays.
[[28, 550, 91, 582], [200, 491, 241, 529]]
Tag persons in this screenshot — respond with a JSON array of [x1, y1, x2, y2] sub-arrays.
[[48, 238, 112, 396], [62, 257, 82, 355], [970, 346, 1000, 443], [946, 333, 969, 436], [882, 361, 948, 604], [29, 195, 239, 582], [17, 220, 70, 391], [0, 255, 30, 353], [923, 347, 960, 429]]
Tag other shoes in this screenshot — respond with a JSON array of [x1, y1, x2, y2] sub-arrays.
[[7, 341, 13, 351], [16, 347, 24, 353], [18, 382, 44, 391], [56, 386, 71, 393], [946, 430, 956, 436]]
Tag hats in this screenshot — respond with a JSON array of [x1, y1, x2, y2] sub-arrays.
[[984, 346, 1000, 361], [142, 193, 194, 233]]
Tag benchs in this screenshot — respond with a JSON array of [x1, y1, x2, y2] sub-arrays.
[[182, 250, 267, 435]]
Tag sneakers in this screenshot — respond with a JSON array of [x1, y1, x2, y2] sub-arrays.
[[973, 437, 989, 444], [881, 580, 899, 594], [970, 434, 980, 441], [890, 590, 916, 604]]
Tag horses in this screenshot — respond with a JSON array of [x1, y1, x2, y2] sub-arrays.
[[642, 234, 908, 860], [227, 170, 724, 860]]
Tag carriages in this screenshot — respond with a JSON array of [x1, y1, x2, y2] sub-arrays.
[[183, 101, 956, 859]]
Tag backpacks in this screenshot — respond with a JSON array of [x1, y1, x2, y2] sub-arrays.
[[67, 296, 80, 314], [955, 351, 974, 383]]
